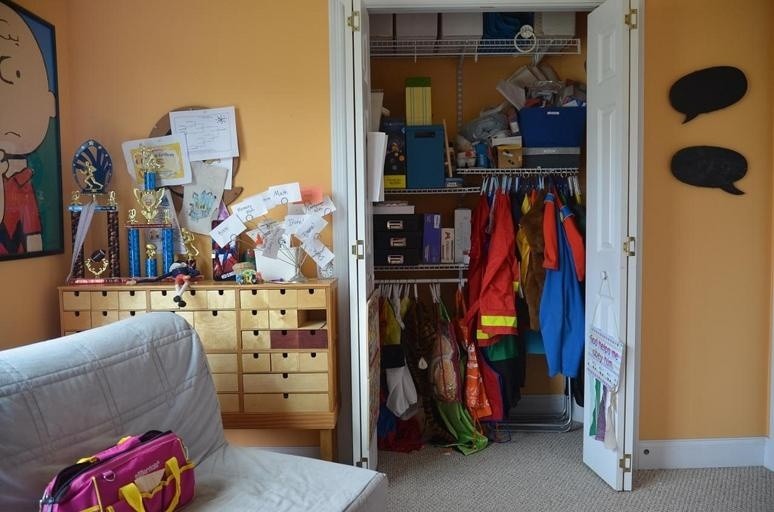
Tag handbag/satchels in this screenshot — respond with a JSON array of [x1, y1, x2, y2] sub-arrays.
[[38, 427, 195, 511]]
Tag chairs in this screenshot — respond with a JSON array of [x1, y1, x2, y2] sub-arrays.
[[482, 331, 573, 433]]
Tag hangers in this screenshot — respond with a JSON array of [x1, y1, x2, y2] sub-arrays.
[[378, 278, 470, 325], [480, 171, 582, 210]]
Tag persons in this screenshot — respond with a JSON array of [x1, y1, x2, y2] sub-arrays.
[[384, 138, 405, 176]]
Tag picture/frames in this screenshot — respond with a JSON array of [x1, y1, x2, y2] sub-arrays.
[[0, 0, 65, 262]]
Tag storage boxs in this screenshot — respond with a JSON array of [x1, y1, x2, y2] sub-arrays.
[[533, 12, 576, 52], [403, 125, 445, 191], [369, 12, 483, 55], [518, 105, 587, 148]]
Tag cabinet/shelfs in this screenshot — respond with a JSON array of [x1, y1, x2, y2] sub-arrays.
[[368, 37, 587, 272]]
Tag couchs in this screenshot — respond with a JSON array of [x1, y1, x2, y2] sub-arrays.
[[0, 310, 390, 512]]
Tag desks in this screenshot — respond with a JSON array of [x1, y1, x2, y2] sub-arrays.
[[57, 276, 341, 462]]
